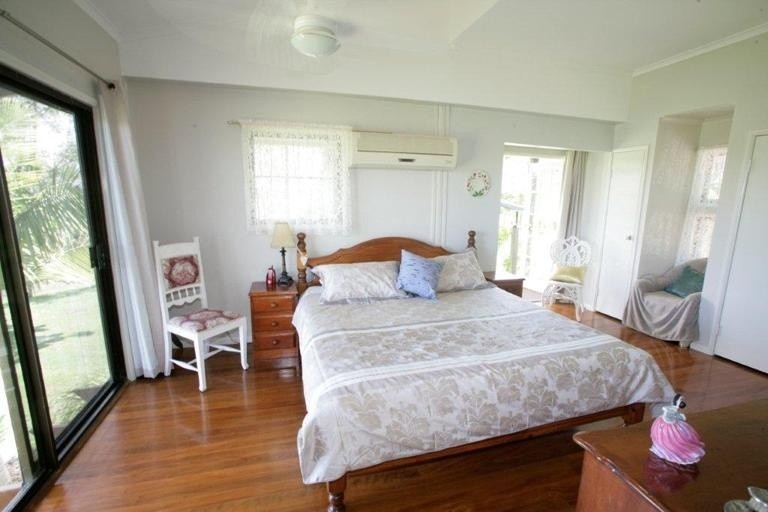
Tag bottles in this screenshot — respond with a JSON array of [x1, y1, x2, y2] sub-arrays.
[[265, 264, 276, 291]]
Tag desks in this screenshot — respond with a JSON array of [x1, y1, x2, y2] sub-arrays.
[[572, 397, 768, 512]]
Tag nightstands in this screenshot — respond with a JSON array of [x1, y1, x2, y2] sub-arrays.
[[248, 279, 300, 371], [482, 271, 526, 298]]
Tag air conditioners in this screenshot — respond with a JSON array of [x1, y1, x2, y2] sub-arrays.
[[351, 129, 457, 171]]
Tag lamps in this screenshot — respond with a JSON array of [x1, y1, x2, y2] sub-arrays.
[[270, 222, 297, 288], [289, 15, 343, 61]]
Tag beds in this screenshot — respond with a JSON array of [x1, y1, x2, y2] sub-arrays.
[[297, 231, 647, 512]]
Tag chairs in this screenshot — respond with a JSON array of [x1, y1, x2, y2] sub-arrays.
[[620, 256, 707, 350], [541, 235, 592, 322], [153, 236, 250, 393]]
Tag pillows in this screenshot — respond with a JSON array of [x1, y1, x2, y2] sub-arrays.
[[550, 265, 586, 285], [310, 248, 488, 303], [665, 265, 705, 298]]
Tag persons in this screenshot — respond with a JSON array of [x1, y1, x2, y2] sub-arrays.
[[649, 393, 706, 466]]
